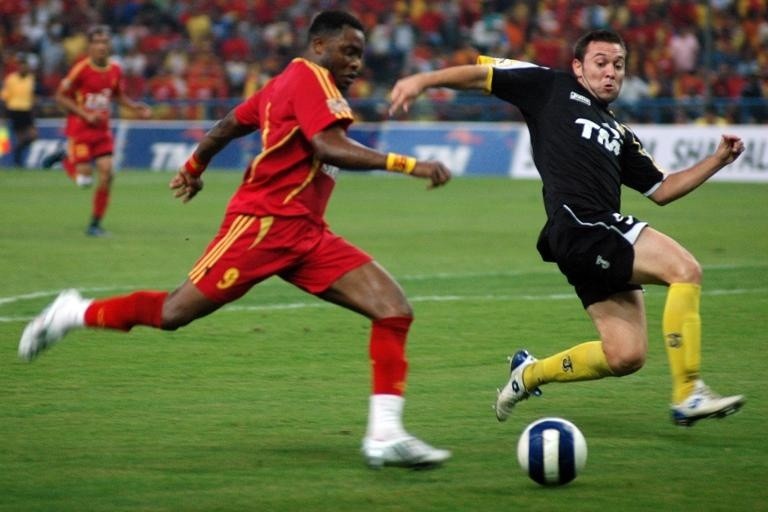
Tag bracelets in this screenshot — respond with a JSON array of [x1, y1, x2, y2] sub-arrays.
[[180, 152, 208, 178], [386, 151, 421, 177]]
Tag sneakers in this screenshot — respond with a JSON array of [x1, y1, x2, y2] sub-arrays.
[[361, 436, 452, 467], [39, 151, 67, 170], [16, 289, 81, 362], [669, 379, 748, 425], [491, 348, 542, 422], [84, 221, 106, 236]]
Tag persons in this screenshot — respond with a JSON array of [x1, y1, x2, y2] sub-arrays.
[[387, 22, 747, 432], [0, 53, 41, 168], [51, 27, 154, 239], [14, 6, 465, 474], [0, 0, 768, 127]]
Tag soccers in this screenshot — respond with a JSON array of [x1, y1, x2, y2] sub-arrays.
[[516, 416, 587, 485]]
